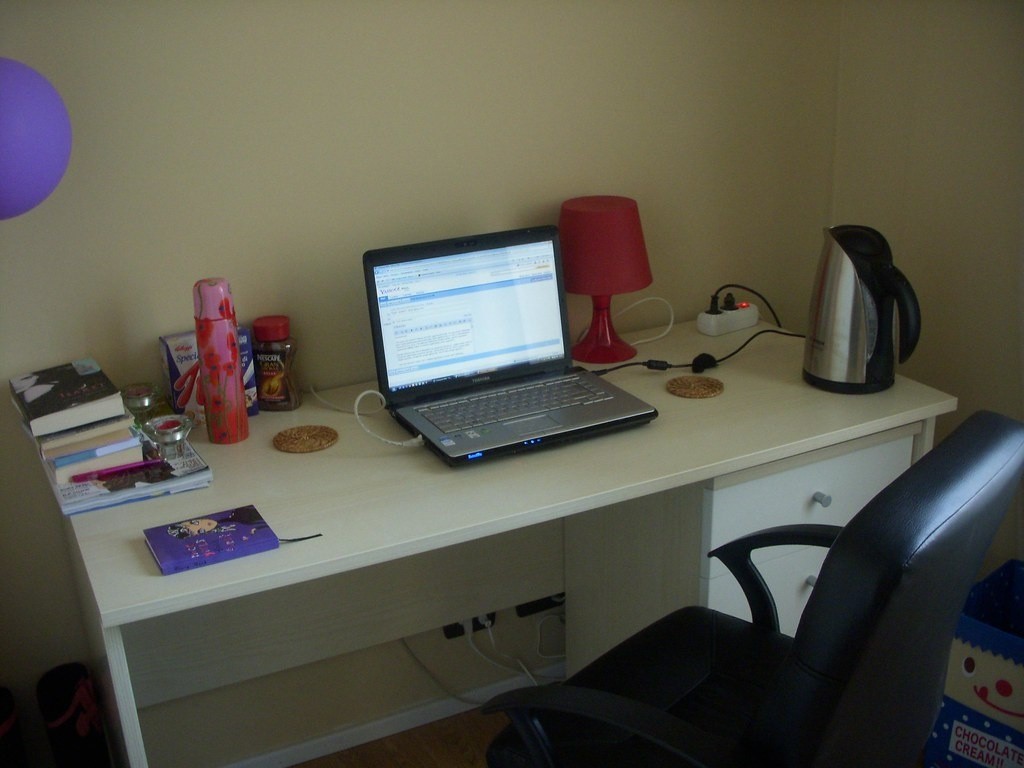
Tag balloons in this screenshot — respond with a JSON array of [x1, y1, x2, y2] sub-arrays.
[[0, 59, 72, 219]]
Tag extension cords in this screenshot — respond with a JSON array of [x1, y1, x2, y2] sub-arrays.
[[696, 302, 758, 336]]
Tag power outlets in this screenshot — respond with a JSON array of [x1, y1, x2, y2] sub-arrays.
[[442, 611, 497, 639], [515, 591, 566, 618]]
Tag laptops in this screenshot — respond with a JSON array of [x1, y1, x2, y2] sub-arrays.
[[363, 225, 658, 466]]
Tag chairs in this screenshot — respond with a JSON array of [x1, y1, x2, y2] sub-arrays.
[[479, 409, 1024, 768]]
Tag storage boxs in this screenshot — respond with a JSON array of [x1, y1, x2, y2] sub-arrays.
[[922, 558, 1024, 768], [158, 322, 259, 428]]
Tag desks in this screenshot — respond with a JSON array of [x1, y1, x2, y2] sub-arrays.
[[71, 315, 957, 768]]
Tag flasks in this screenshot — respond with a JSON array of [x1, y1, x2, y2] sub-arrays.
[[192, 277, 249, 445]]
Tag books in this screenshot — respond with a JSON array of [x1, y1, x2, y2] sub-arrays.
[[8, 357, 214, 517], [143, 504, 323, 577]]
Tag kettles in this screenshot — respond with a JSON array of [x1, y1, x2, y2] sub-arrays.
[[802, 225, 921, 395]]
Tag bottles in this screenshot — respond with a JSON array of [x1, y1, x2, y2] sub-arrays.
[[252, 316, 305, 411]]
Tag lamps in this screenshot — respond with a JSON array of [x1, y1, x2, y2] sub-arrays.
[[559, 195, 655, 363]]
